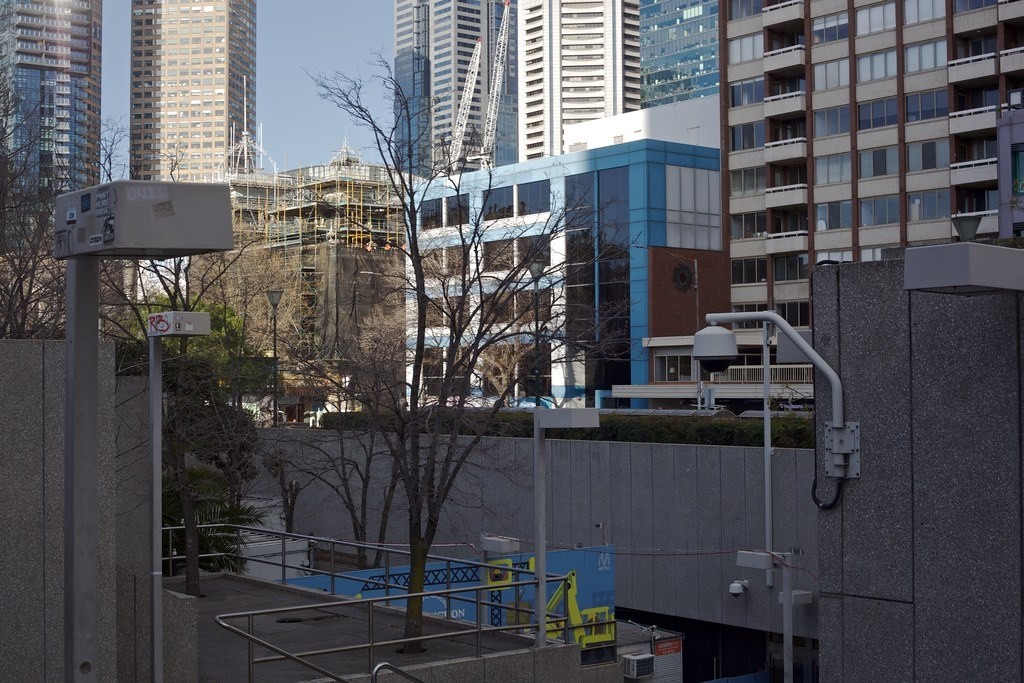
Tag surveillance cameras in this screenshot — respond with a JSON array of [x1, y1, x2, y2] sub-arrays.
[[730, 583, 743, 597], [692, 324, 738, 373]]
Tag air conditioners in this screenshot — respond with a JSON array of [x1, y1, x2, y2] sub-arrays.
[[619, 653, 656, 680]]
